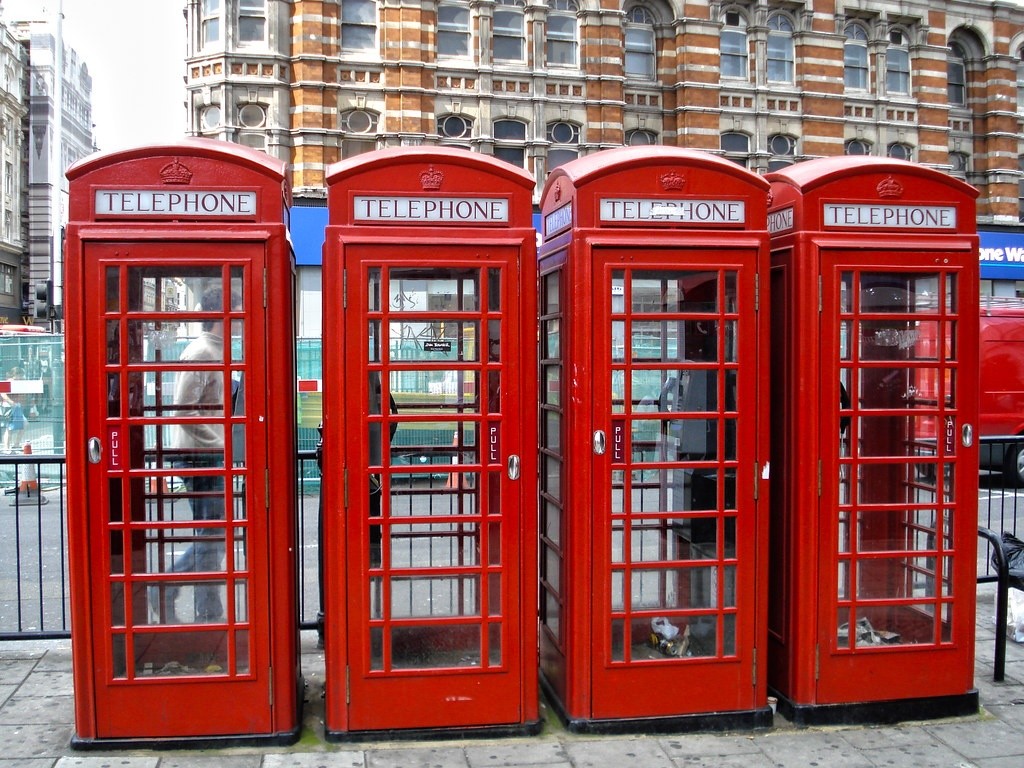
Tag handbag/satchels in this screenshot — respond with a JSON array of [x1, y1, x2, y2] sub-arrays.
[[7, 403, 25, 430]]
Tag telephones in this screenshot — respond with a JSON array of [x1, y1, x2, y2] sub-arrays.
[[656, 359, 708, 456], [368, 370, 399, 468], [840, 382, 852, 435], [228, 371, 246, 464]]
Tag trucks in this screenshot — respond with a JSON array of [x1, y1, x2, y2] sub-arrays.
[[914, 306, 1023, 487]]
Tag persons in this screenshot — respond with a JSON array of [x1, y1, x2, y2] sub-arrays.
[[147, 286, 243, 623], [0, 366, 29, 456]]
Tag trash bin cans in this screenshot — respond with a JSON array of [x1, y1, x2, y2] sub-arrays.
[[990, 531, 1024, 643]]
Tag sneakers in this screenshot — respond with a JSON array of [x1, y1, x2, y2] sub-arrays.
[[144, 586, 180, 626], [191, 613, 234, 624]]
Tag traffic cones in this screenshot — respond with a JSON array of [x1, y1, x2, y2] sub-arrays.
[[443, 431, 470, 492], [9, 441, 49, 506], [141, 442, 182, 504]]
